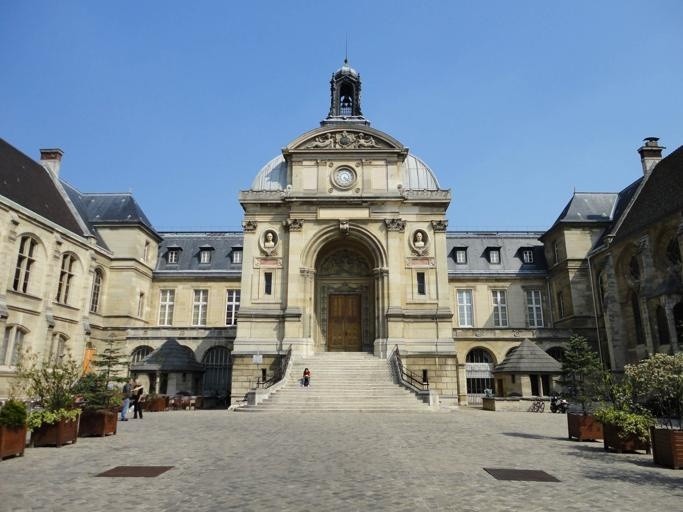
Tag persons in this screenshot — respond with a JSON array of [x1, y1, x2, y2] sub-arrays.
[[415, 232, 424, 247], [338, 130, 351, 149], [306, 133, 333, 149], [265, 233, 274, 247], [132, 380, 143, 418], [302, 368, 311, 387], [354, 133, 382, 147], [121, 378, 134, 421]]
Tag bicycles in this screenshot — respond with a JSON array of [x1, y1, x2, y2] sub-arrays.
[[532, 400, 545, 413]]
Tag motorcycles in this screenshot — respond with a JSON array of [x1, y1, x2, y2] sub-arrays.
[[548, 396, 568, 413]]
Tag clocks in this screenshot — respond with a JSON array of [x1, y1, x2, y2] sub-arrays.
[[328, 164, 358, 190]]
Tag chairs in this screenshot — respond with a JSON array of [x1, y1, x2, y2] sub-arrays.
[[142, 391, 204, 412]]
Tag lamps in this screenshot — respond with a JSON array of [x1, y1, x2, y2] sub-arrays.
[[252, 350, 264, 383]]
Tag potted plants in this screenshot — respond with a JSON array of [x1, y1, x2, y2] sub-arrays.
[[592, 369, 653, 454], [0, 396, 25, 461], [621, 348, 683, 470], [551, 331, 605, 444], [481, 388, 495, 411], [76, 330, 124, 439], [19, 343, 80, 446]]
